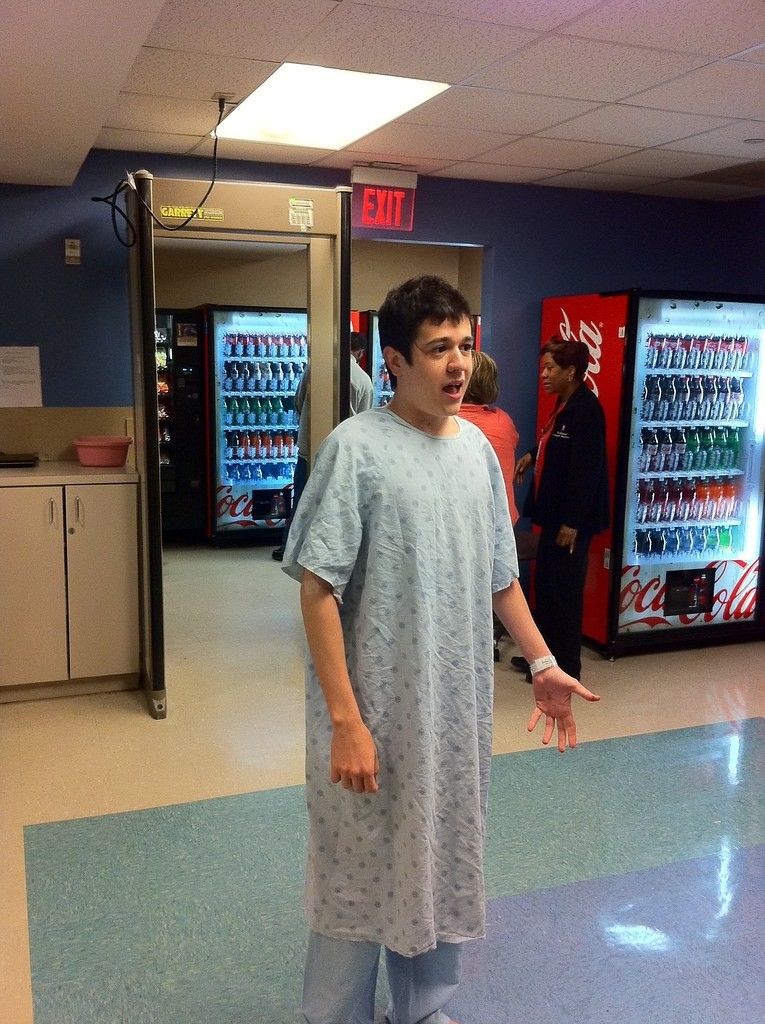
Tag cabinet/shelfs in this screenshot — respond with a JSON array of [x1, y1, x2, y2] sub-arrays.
[[0, 459, 141, 705]]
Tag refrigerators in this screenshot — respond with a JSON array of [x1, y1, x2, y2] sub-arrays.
[[528, 285, 765, 662], [171, 302, 309, 551], [349, 309, 481, 419]]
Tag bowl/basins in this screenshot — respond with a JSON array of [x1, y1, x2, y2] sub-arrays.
[[74, 435, 133, 467]]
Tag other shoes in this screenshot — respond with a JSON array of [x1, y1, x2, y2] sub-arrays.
[[510, 655, 581, 684], [272, 548, 284, 561]]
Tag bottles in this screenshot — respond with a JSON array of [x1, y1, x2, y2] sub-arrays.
[[380, 361, 395, 407], [626, 335, 756, 558], [222, 333, 310, 482]]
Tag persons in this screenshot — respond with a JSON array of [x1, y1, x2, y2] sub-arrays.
[[273, 331, 374, 562], [456, 350, 521, 529], [510, 341, 612, 682], [281, 274, 600, 1024]]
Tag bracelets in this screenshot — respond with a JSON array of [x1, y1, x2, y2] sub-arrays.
[[529, 655, 557, 677]]
